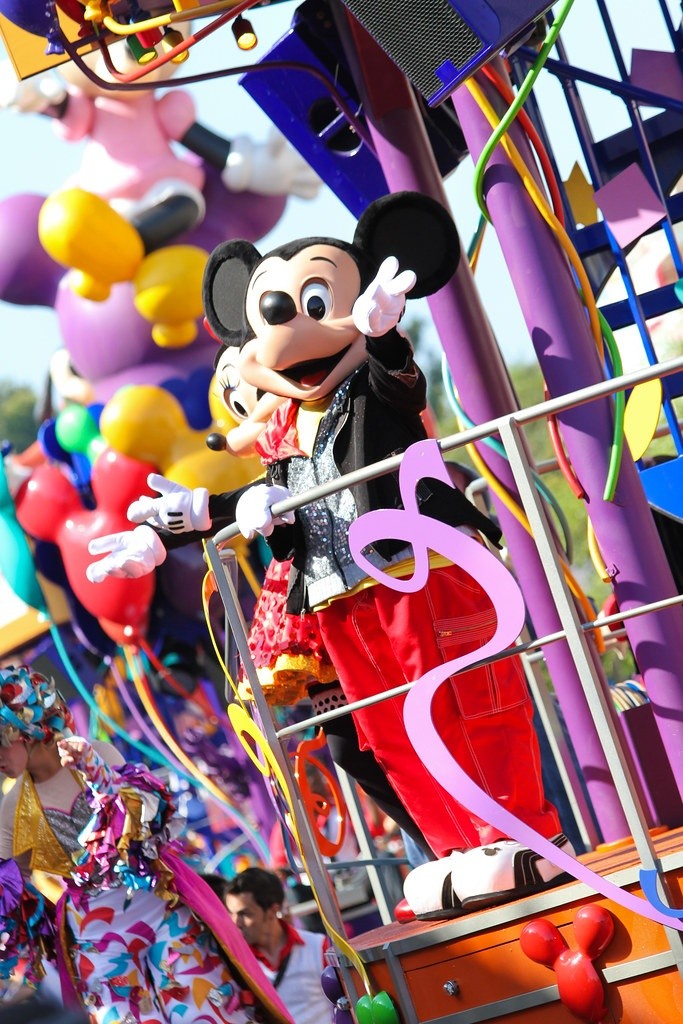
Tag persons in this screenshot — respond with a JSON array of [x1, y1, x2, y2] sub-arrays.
[[222, 868, 337, 1024], [1, 664, 297, 1024]]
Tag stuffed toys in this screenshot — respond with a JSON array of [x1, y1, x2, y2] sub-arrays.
[[0, 16, 322, 251], [200, 190, 576, 921], [85, 316, 418, 924]]
